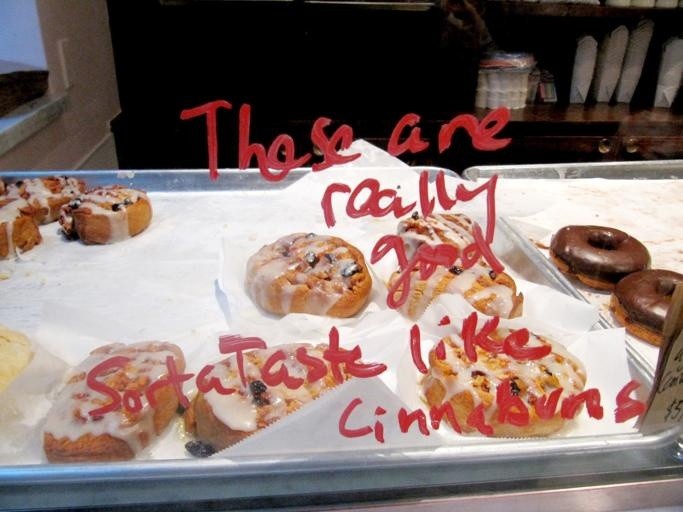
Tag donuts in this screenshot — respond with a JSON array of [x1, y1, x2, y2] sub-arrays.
[[550, 225, 650, 289], [611, 269, 683, 347]]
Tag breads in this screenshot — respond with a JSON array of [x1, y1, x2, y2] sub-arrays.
[[421, 327, 587, 437], [244, 233, 372, 318], [183, 343, 363, 458], [0, 176, 152, 259], [385, 259, 524, 325], [395, 214, 481, 262], [0, 326, 33, 394], [43, 340, 184, 461]]
